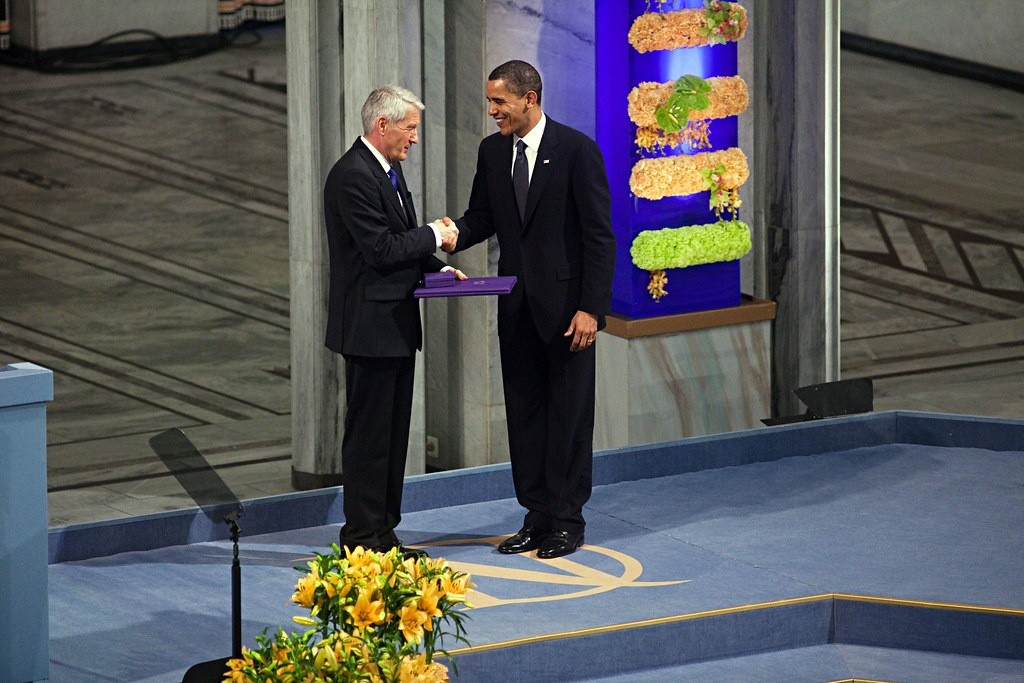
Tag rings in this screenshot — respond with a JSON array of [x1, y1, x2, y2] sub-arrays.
[[586, 339, 594, 343]]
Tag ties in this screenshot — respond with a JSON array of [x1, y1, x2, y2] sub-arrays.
[[513, 139, 529, 224], [388, 166, 399, 192]]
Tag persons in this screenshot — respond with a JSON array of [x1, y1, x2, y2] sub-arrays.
[[440, 60, 618, 559], [323, 84, 468, 560]]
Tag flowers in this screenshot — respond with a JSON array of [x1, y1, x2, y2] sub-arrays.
[[697, 0, 741, 47], [702, 166, 744, 222], [648, 270, 669, 301], [635, 72, 713, 159], [219, 540, 479, 683]]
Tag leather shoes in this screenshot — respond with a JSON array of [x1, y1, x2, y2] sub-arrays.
[[537, 528, 584, 558], [341, 543, 429, 563], [497, 521, 545, 554]]
